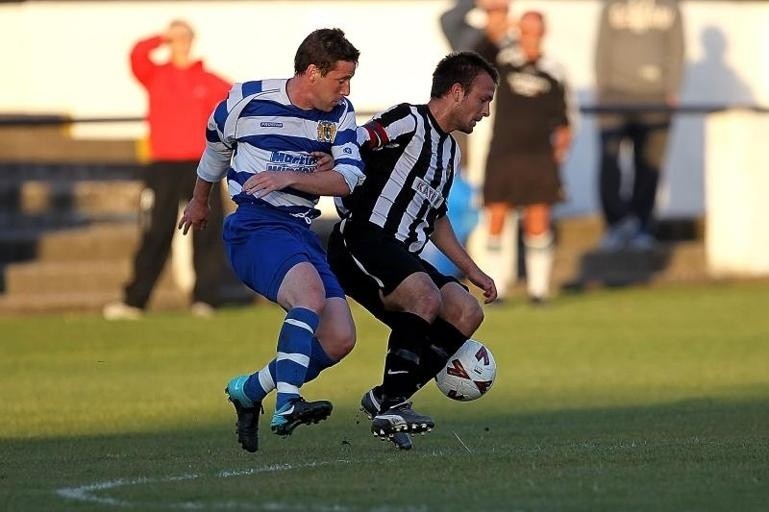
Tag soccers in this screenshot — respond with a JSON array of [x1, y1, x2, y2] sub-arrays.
[[435, 339, 496, 401]]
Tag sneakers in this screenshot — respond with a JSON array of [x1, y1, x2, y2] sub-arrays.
[[358, 385, 413, 450], [225, 373, 264, 452], [371, 405, 435, 437], [270, 396, 332, 440], [103, 300, 215, 322]]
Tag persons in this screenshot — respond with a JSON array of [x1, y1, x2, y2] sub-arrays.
[[439, 1, 515, 206], [309, 50, 498, 450], [481, 10, 573, 301], [178, 28, 367, 454], [591, 0, 685, 226], [101, 19, 238, 321]]
